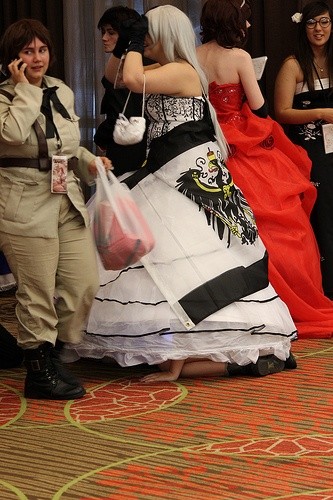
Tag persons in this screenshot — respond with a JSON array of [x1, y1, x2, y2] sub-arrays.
[[0, 0, 333, 383], [0, 20, 114, 401]]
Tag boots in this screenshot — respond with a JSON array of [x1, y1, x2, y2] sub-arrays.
[[23, 341, 86, 400]]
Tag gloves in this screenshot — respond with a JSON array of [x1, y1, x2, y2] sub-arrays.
[[113, 14, 148, 58]]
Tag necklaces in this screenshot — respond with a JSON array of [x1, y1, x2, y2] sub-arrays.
[[314, 56, 329, 72]]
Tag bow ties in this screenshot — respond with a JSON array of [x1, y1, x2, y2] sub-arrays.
[[41, 86, 72, 138]]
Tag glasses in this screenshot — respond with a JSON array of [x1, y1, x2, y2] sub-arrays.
[[306, 17, 332, 28]]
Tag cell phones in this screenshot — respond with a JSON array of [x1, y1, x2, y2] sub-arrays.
[[13, 55, 26, 74]]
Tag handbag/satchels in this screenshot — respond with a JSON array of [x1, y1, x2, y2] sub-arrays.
[[89, 156, 157, 271], [113, 113, 146, 146]]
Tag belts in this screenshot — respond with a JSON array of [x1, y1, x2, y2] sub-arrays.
[[0, 157, 81, 173]]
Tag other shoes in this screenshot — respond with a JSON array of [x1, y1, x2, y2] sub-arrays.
[[248, 354, 286, 376], [283, 350, 297, 368]]
